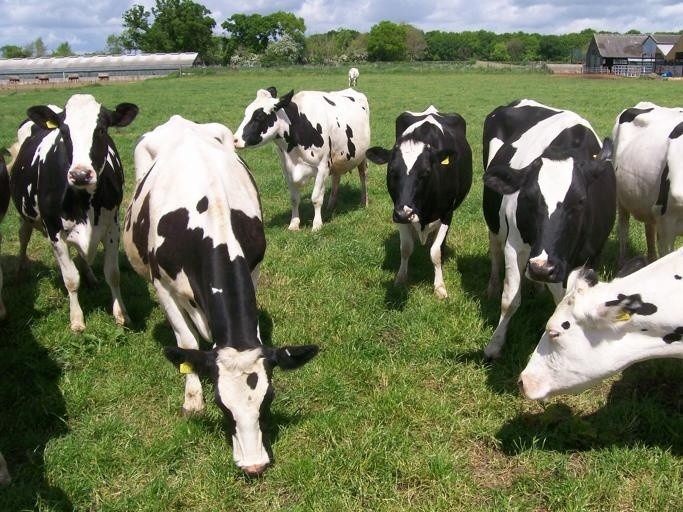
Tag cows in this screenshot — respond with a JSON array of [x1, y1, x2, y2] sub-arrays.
[[348, 67, 359, 89], [364, 103, 473, 302], [232, 85, 372, 234], [612, 100, 683, 264], [121, 111, 321, 481], [516, 247, 683, 403], [7, 92, 140, 336], [476, 97, 618, 367]]
